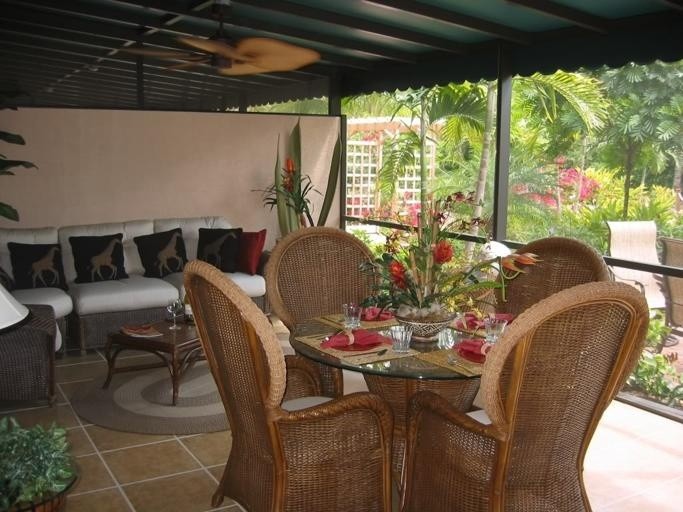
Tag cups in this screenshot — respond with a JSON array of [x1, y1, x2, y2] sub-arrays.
[[167, 298, 184, 331], [390, 325, 414, 354], [484, 318, 508, 343], [342, 303, 362, 328]]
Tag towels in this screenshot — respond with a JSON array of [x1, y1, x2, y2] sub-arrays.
[[454, 338, 493, 364], [458, 310, 513, 330], [348, 301, 395, 321], [320, 328, 384, 349]]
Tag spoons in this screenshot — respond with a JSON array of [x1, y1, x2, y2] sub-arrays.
[[344, 349, 387, 358], [447, 352, 476, 376]]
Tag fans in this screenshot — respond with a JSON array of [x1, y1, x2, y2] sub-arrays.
[[108, 2, 321, 77]]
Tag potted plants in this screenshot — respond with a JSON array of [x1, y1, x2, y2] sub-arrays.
[[0, 415, 82, 511]]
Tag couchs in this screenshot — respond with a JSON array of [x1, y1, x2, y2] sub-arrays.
[[58, 214, 266, 357], [0, 214, 75, 411]]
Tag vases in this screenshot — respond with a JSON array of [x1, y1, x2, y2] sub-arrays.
[[392, 311, 458, 343]]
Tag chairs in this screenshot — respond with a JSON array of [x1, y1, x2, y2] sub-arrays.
[[652, 236, 683, 355], [604, 220, 679, 347], [181, 259, 393, 511], [492, 236, 613, 324], [400, 281, 650, 512], [264, 228, 380, 399]]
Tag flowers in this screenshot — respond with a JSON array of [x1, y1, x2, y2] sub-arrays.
[[251, 155, 324, 228], [361, 192, 544, 317]]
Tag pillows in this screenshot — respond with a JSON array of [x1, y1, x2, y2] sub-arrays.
[[7, 242, 69, 293], [133, 228, 188, 278], [197, 228, 243, 273], [68, 233, 130, 284], [238, 229, 267, 276]]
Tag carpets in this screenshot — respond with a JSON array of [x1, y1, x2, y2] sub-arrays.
[[72, 331, 296, 435]]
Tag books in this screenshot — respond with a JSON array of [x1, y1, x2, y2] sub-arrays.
[[122, 324, 163, 338]]
